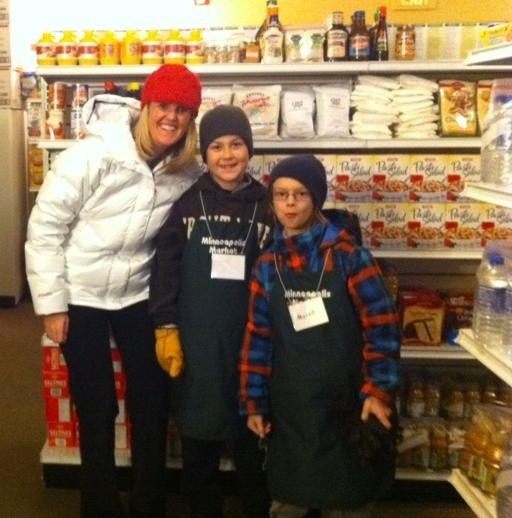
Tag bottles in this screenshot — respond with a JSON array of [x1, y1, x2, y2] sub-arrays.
[[254, 0, 285, 64], [323, 5, 390, 62], [474, 248, 512, 351]]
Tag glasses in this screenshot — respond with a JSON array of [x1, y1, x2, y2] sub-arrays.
[[273, 188, 310, 200]]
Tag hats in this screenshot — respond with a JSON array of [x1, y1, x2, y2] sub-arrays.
[[200, 105, 253, 164], [142, 65, 201, 112], [270, 154, 326, 206]]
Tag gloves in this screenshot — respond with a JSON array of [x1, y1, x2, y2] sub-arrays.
[[154, 326, 184, 378]]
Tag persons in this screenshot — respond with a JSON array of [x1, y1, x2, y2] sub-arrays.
[[235, 153, 402, 517], [24, 63, 206, 518], [143, 105, 279, 517]]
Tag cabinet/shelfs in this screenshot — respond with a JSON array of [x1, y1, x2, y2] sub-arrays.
[[19, 42, 512, 518]]
